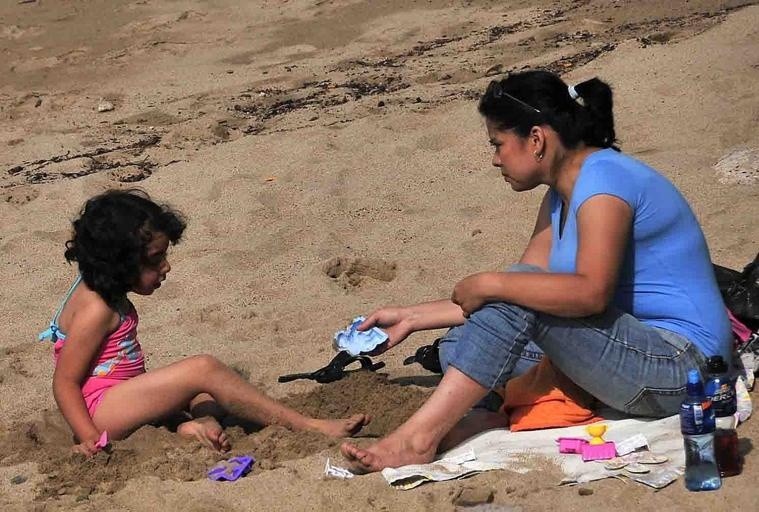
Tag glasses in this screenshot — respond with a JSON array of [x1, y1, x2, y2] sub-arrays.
[[486, 80, 541, 113]]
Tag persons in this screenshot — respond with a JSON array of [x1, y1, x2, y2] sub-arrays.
[[37, 187, 373, 458], [337, 64, 738, 473]]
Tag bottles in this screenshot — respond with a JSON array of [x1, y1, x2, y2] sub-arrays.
[[704, 356, 744, 479], [679, 371, 722, 491]]
